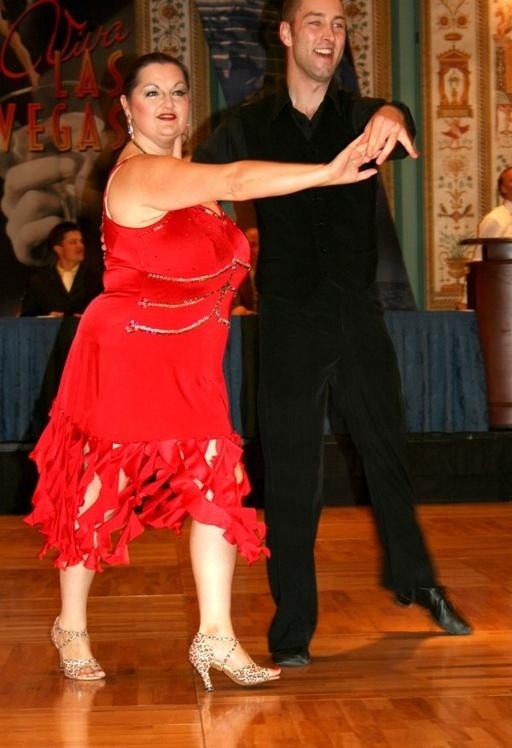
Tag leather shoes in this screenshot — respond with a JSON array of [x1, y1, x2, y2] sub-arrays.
[[396, 586, 472, 635], [272, 648, 311, 666]]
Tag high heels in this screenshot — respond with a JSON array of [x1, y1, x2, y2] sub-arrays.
[[187, 632, 281, 691], [50, 616, 106, 680]]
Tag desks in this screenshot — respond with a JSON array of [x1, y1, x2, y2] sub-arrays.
[[1, 310, 487, 453]]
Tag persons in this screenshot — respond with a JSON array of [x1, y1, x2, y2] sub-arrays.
[[1, 110, 117, 269], [24, 51, 385, 690], [21, 221, 97, 318], [52, 681, 280, 748], [231, 228, 260, 316], [101, 0, 473, 668], [458, 168, 512, 310]]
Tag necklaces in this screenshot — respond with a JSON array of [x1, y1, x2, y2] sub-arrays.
[[132, 140, 146, 154]]
